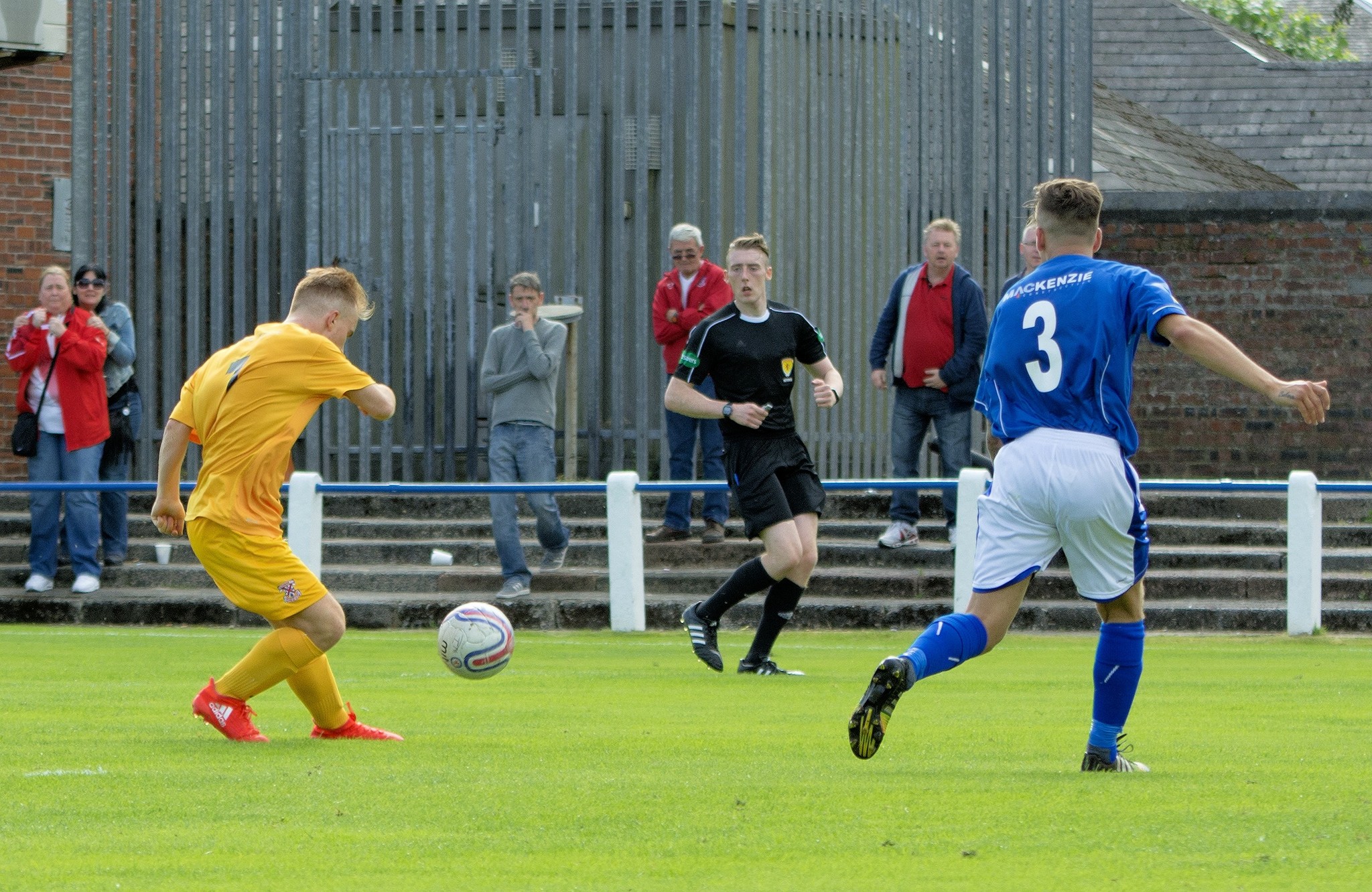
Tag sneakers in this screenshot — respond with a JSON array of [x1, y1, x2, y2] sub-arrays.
[[1081, 734, 1149, 773], [192, 677, 267, 743], [25, 571, 53, 592], [540, 542, 569, 573], [646, 524, 692, 543], [495, 579, 530, 599], [737, 658, 803, 677], [72, 573, 100, 593], [848, 658, 909, 759], [681, 602, 723, 673], [104, 555, 122, 566], [702, 522, 725, 543], [57, 553, 72, 565], [310, 701, 403, 740]]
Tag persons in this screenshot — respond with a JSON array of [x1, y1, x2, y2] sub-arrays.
[[665, 230, 844, 675], [869, 221, 989, 549], [480, 272, 568, 598], [5, 266, 111, 593], [151, 266, 404, 741], [848, 179, 1330, 773], [645, 223, 734, 543], [14, 263, 143, 565], [1002, 214, 1042, 298]]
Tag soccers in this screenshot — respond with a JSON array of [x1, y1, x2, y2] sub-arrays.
[[439, 602, 516, 679]]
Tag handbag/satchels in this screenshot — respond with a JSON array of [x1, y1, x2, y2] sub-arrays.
[[12, 411, 38, 456]]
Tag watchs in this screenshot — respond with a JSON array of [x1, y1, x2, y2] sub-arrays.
[[831, 389, 840, 406], [672, 313, 677, 322], [723, 402, 734, 419]]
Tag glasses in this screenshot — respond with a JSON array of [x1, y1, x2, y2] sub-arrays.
[[77, 279, 104, 290]]
